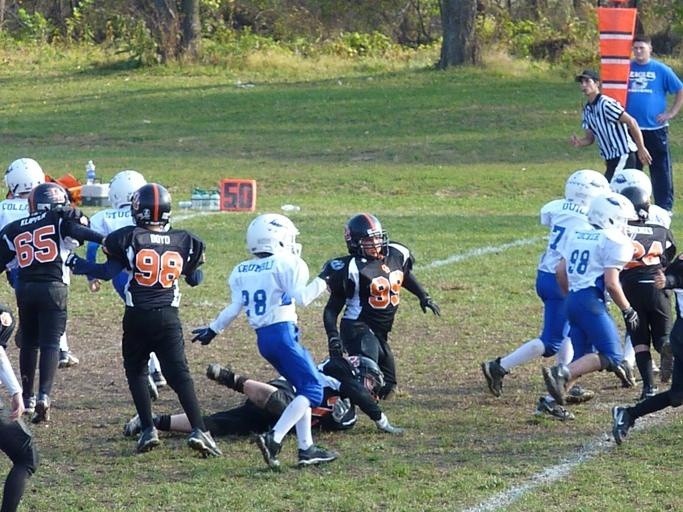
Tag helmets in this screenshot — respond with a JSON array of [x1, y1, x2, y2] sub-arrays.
[[6, 157, 45, 197], [564, 168, 653, 233], [247, 213, 302, 256], [347, 356, 385, 396], [345, 213, 389, 261], [132, 183, 173, 225], [108, 171, 147, 207], [28, 183, 71, 214]]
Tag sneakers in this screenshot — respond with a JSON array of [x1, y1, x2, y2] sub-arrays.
[[208, 364, 236, 384], [482, 357, 508, 397], [123, 369, 168, 451], [23, 392, 50, 423], [612, 406, 635, 445], [58, 348, 80, 368], [188, 427, 221, 458], [614, 341, 676, 400], [297, 445, 340, 467], [537, 363, 593, 420], [257, 429, 283, 469]]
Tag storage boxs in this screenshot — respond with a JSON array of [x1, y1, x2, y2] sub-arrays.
[[79, 183, 110, 207]]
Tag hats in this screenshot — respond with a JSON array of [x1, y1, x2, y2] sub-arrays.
[[575, 70, 601, 86]]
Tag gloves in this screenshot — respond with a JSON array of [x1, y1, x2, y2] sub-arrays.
[[328, 334, 345, 358], [384, 422, 411, 433], [191, 328, 216, 346], [319, 260, 346, 284], [66, 253, 78, 265], [421, 298, 442, 316], [622, 307, 639, 333], [333, 397, 353, 419]]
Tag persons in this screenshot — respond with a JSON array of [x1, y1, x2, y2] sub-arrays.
[[0, 302, 40, 511], [86, 169, 168, 401], [0, 183, 108, 423], [483, 168, 682, 445], [626, 35, 683, 217], [0, 158, 83, 369], [123, 355, 405, 435], [189, 214, 340, 469], [571, 68, 653, 186], [321, 212, 441, 399], [65, 182, 224, 454]]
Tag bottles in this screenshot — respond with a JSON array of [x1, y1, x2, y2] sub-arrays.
[[86, 159, 96, 185], [280, 204, 301, 212], [191, 187, 220, 211], [178, 201, 193, 207]]
